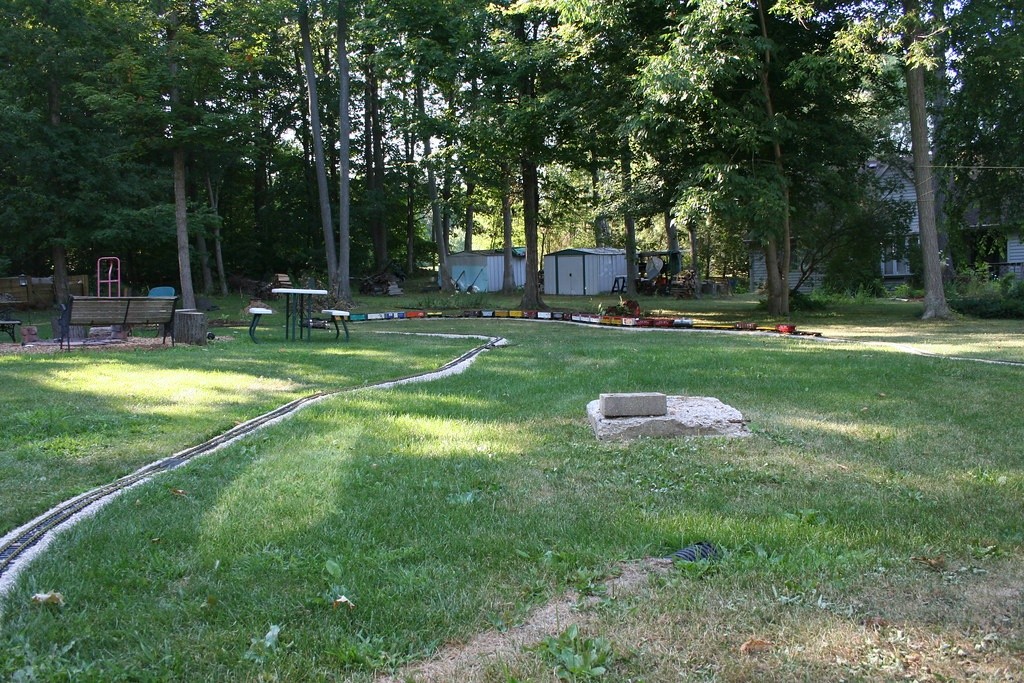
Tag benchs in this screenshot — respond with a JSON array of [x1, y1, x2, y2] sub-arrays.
[[0, 302, 23, 343], [57, 294, 179, 355], [322, 310, 349, 343], [249, 308, 272, 345]]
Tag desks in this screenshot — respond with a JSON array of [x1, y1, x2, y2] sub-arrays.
[[271, 288, 328, 344]]
